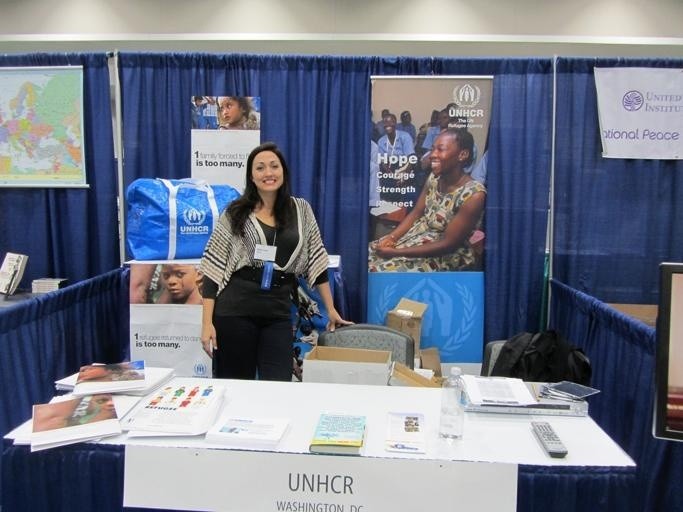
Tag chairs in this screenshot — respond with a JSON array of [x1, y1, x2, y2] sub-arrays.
[[317, 323, 414, 370]]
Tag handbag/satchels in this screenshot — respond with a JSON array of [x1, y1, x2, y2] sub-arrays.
[[124, 177, 243, 261]]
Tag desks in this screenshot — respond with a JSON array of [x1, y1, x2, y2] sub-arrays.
[[0, 376, 638, 511]]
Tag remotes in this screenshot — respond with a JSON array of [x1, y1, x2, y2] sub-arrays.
[[532, 419, 568, 458]]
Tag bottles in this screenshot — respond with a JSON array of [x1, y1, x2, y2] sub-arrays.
[[440, 367, 467, 440]]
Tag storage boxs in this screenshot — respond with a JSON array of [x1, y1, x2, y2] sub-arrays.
[[388, 299, 427, 357], [303, 346, 392, 384], [388, 364, 436, 388]]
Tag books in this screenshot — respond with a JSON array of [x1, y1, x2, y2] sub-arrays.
[[73, 359, 145, 399], [0, 251, 30, 296], [31, 277, 68, 293], [26, 396, 121, 453], [309, 414, 368, 458]]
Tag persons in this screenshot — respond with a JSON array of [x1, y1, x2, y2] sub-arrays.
[[199, 142, 356, 381], [368, 106, 489, 273], [191, 96, 260, 130], [154, 262, 202, 306]]
